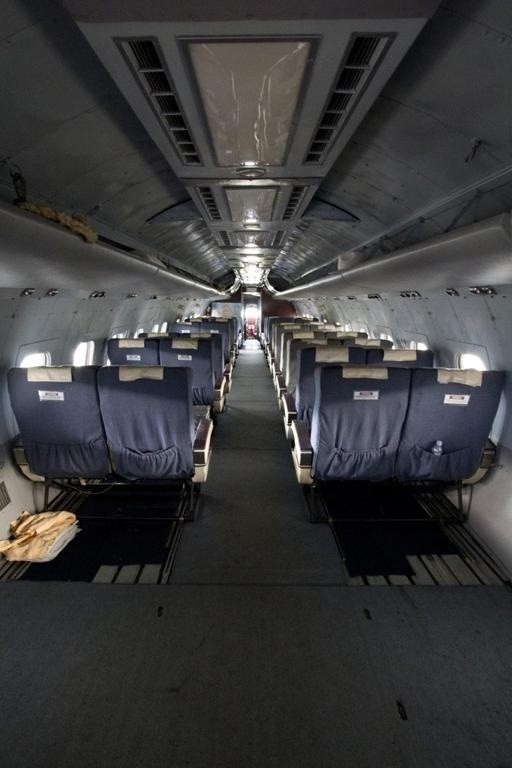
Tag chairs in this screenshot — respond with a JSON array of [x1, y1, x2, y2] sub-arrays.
[[0, 311, 244, 524]]
